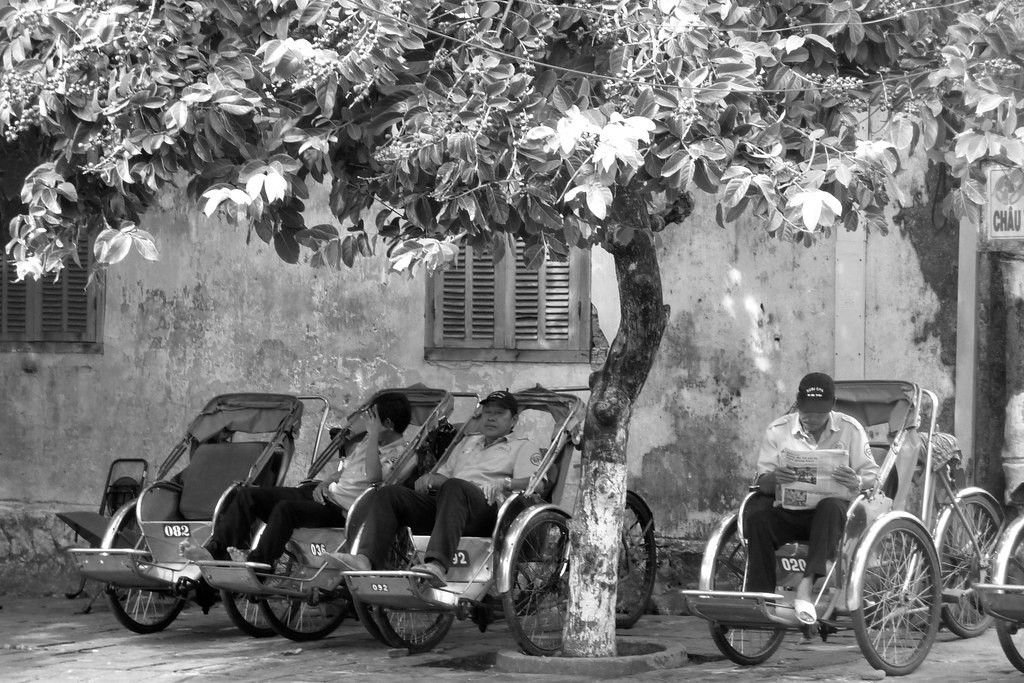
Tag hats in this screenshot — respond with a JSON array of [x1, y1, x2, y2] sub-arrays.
[[480, 388, 517, 414], [796, 372, 835, 413]]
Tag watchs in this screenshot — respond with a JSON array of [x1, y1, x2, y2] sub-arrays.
[[505, 477, 511, 490]]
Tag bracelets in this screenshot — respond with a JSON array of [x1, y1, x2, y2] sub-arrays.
[[856, 475, 864, 493]]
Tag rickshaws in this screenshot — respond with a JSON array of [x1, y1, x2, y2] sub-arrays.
[[681, 378, 1007, 677], [68, 391, 329, 638], [337, 383, 659, 657], [974, 474, 1023, 676], [189, 384, 483, 645]]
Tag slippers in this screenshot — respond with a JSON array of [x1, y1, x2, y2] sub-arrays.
[[410, 562, 448, 587], [321, 552, 357, 571], [794, 599, 817, 625]]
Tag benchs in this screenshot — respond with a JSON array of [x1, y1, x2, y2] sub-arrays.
[[401, 461, 558, 583], [134, 439, 285, 562], [773, 444, 898, 561], [289, 525, 352, 572]]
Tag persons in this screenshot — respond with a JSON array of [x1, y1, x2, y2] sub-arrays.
[[331, 390, 547, 589], [178, 386, 418, 585], [738, 372, 879, 625]]
